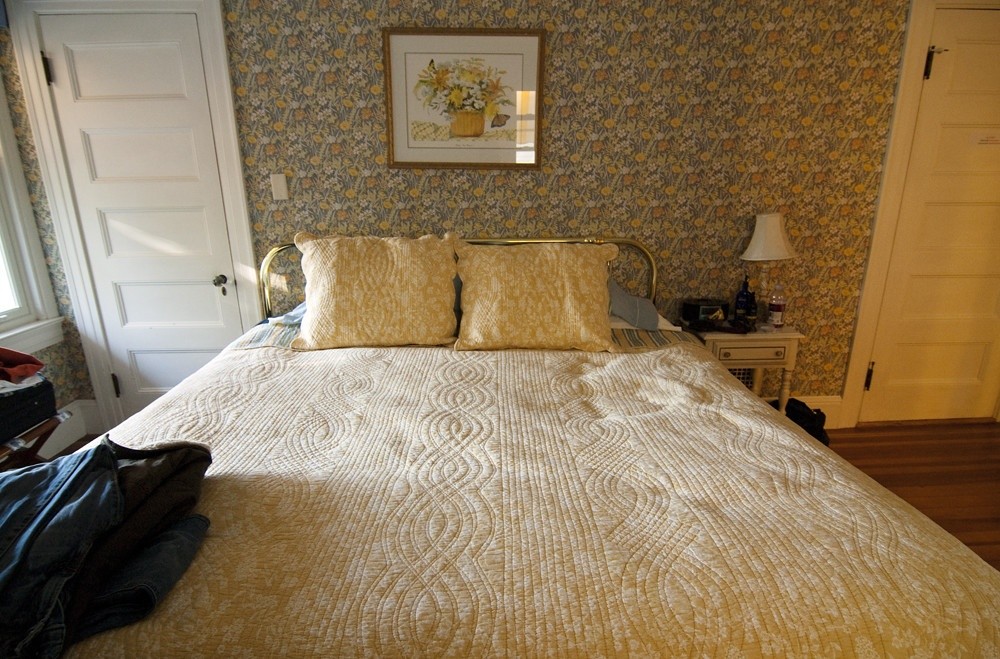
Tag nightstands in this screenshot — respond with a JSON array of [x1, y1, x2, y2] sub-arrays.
[[692, 319, 806, 413]]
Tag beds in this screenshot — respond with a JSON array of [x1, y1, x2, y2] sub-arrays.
[[59, 244, 1000, 659]]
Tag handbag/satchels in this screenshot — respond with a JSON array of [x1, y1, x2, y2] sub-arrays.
[[769, 398, 831, 449]]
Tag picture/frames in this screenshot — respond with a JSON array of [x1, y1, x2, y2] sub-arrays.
[[381, 26, 544, 171]]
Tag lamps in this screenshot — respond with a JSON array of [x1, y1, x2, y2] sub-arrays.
[[739, 212, 797, 324]]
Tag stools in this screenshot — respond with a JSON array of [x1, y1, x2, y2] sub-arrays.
[[0, 410, 69, 474]]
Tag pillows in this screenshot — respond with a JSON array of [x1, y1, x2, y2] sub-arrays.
[[290, 230, 459, 348], [451, 236, 622, 353]]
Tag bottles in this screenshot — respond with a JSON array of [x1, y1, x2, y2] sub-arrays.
[[734, 282, 758, 326], [769, 285, 786, 328]]
